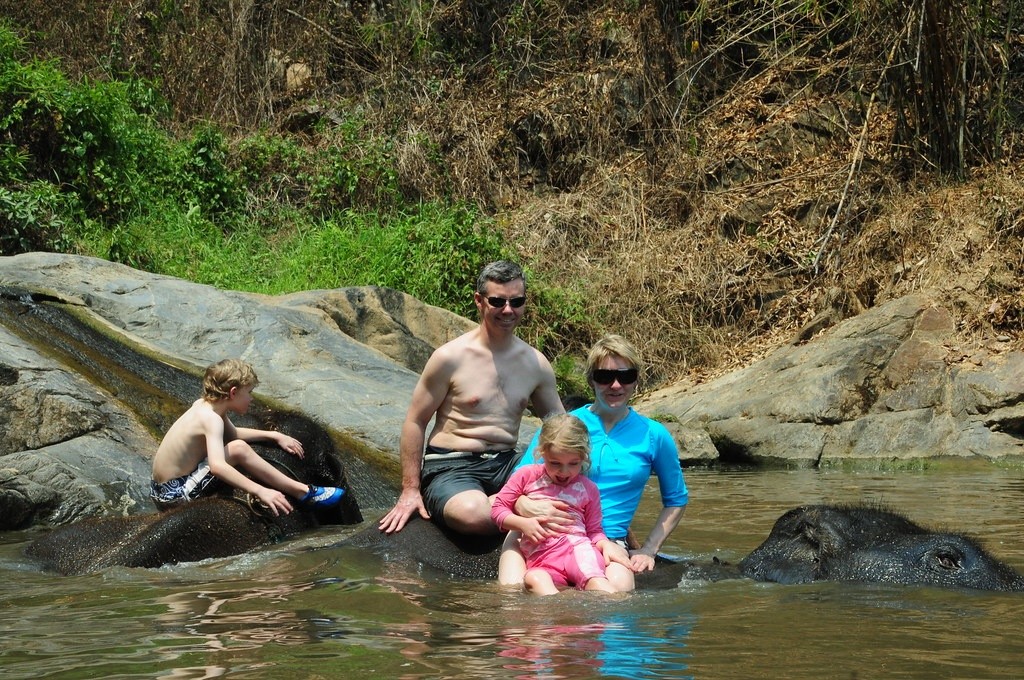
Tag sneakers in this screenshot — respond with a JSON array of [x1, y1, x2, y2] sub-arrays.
[[298, 484, 346, 506]]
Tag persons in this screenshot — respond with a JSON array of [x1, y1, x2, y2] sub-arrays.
[[491, 414, 636, 598], [379, 259, 567, 534], [150, 359, 345, 517], [499, 334, 689, 593]]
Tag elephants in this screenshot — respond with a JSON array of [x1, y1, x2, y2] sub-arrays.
[[25, 407, 1024, 595]]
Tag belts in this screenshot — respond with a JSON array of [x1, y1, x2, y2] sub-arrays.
[[425, 448, 515, 460]]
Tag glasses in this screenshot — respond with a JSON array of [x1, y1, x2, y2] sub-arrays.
[[482, 294, 526, 308], [593, 368, 638, 384]]
